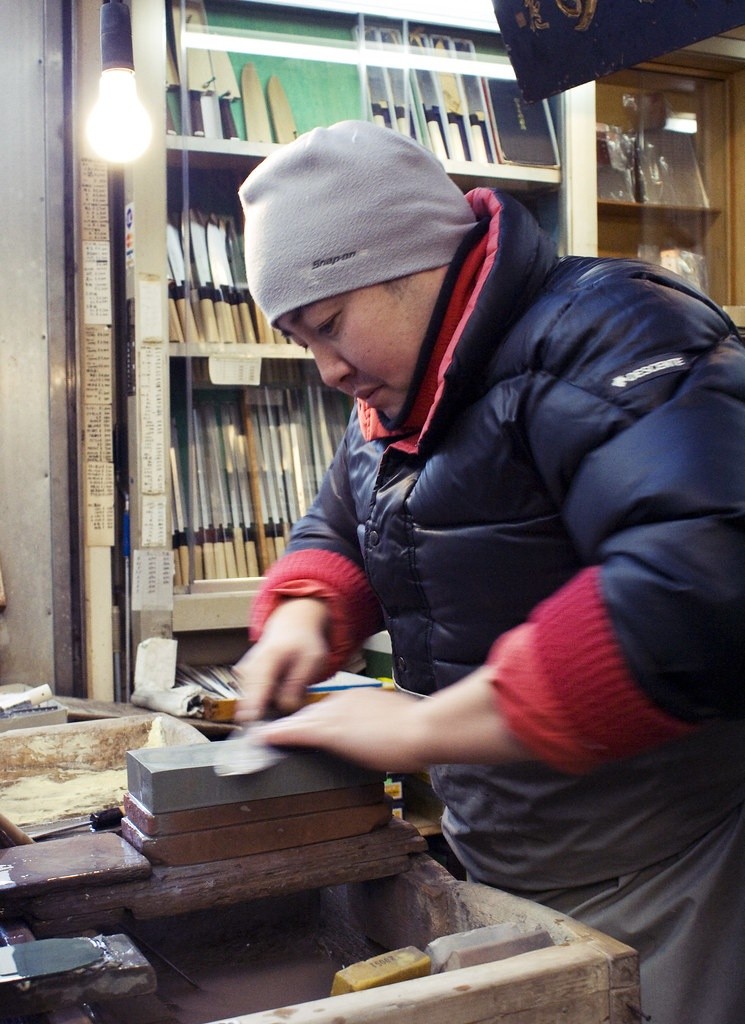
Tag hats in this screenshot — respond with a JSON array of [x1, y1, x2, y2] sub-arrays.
[[237, 119, 479, 329]]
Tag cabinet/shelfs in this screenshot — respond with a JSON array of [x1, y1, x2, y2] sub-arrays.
[[67, 0, 745, 831]]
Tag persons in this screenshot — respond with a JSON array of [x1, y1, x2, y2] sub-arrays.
[[233, 118, 745, 1024]]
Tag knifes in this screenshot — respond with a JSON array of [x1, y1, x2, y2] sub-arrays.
[[165, 0, 497, 589], [214, 712, 291, 775], [27, 806, 127, 838]]
